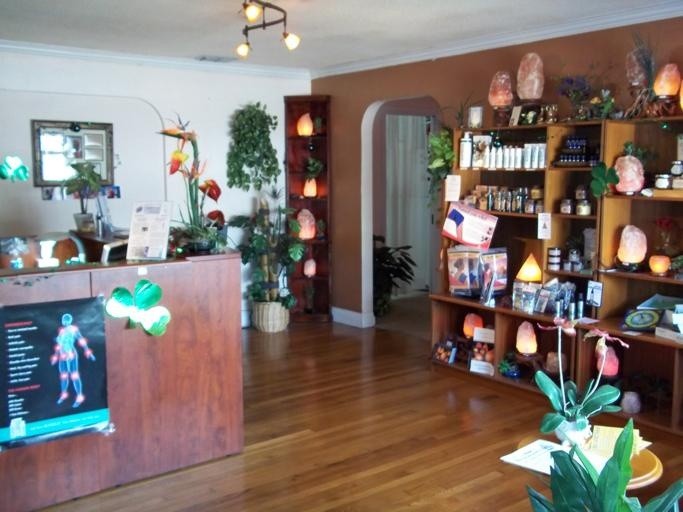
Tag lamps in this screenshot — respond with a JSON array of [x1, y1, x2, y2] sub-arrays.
[[234, 0, 301, 59]]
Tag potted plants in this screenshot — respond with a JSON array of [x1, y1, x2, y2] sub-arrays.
[[59, 159, 108, 234], [225, 99, 307, 335], [373, 231, 417, 317]]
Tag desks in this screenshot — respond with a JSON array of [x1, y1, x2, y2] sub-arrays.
[[517, 432, 664, 491]]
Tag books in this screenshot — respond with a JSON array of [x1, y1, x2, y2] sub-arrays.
[[498, 436, 565, 476]]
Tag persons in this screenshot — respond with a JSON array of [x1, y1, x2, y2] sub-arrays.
[[49, 313, 96, 410], [451, 258, 468, 287]]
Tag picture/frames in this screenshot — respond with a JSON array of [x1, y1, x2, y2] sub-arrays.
[[29, 117, 115, 188]]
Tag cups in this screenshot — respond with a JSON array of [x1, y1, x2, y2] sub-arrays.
[[546, 351, 567, 373]]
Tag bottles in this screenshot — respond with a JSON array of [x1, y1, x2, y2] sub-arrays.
[[484, 144, 545, 170], [559, 135, 598, 169], [620, 391, 641, 414], [553, 292, 585, 321], [589, 289, 593, 304], [486, 186, 529, 215]]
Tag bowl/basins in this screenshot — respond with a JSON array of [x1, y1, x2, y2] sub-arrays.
[[623, 448, 664, 497]]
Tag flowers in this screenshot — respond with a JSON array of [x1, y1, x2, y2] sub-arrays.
[[588, 93, 616, 117], [157, 105, 227, 247], [532, 314, 631, 435], [553, 67, 592, 119]]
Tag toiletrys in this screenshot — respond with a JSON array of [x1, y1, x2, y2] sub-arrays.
[[487, 184, 528, 215], [458, 129, 545, 168]]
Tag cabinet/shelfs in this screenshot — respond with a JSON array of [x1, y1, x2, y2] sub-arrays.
[[427, 113, 682, 438], [282, 93, 332, 324]]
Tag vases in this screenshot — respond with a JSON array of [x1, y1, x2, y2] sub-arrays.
[[550, 416, 592, 445], [185, 236, 215, 254]]
